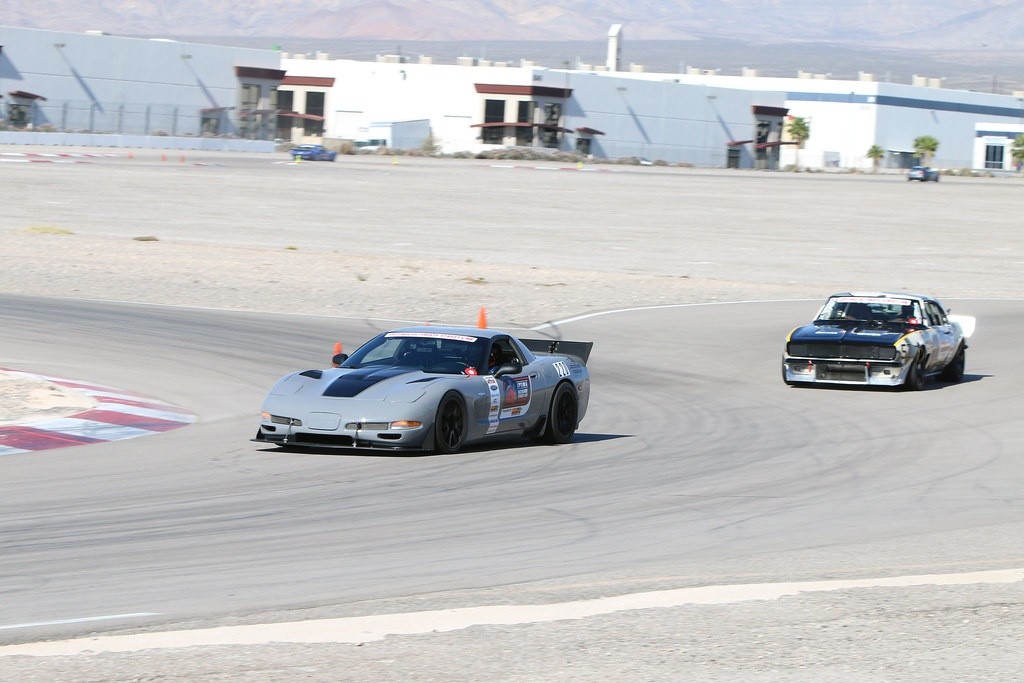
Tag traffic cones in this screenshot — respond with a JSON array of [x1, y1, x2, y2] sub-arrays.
[[328, 340, 343, 367], [475, 306, 487, 330]]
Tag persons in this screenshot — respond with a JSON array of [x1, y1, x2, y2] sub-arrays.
[[488, 349, 500, 375], [894, 300, 918, 323]]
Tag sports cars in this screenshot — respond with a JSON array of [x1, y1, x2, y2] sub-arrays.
[[250, 325, 594, 455], [782, 292, 977, 391]]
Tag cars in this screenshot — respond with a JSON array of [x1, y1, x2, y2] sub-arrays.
[[291, 143, 337, 162], [909, 166, 940, 183]]
